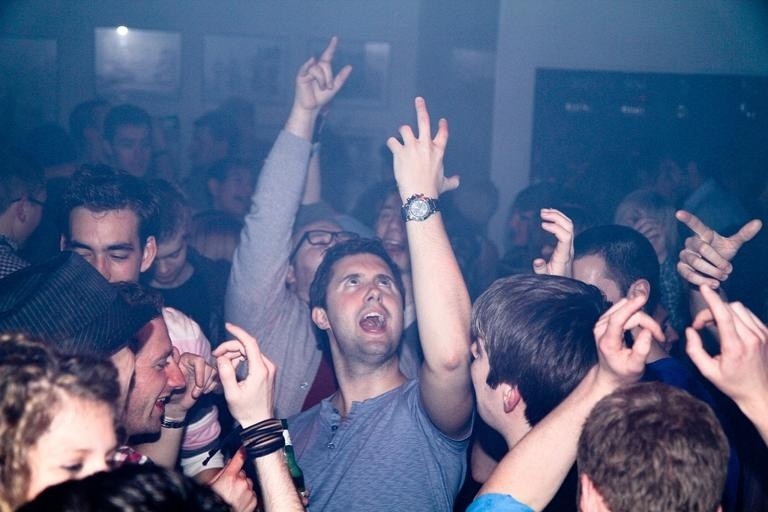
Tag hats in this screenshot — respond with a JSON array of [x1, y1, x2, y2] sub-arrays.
[[0, 251, 159, 359]]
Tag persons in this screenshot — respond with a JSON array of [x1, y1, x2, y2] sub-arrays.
[[226, 33, 480, 512], [0, 94, 768, 511]]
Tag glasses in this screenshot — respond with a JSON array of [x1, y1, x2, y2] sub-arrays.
[[290, 230, 359, 262]]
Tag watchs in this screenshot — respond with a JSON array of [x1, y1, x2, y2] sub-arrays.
[[394, 193, 443, 222]]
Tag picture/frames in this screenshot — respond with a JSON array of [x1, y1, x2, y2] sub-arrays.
[[308, 34, 393, 109], [199, 29, 292, 110], [89, 23, 184, 103], [0, 35, 63, 113]]
